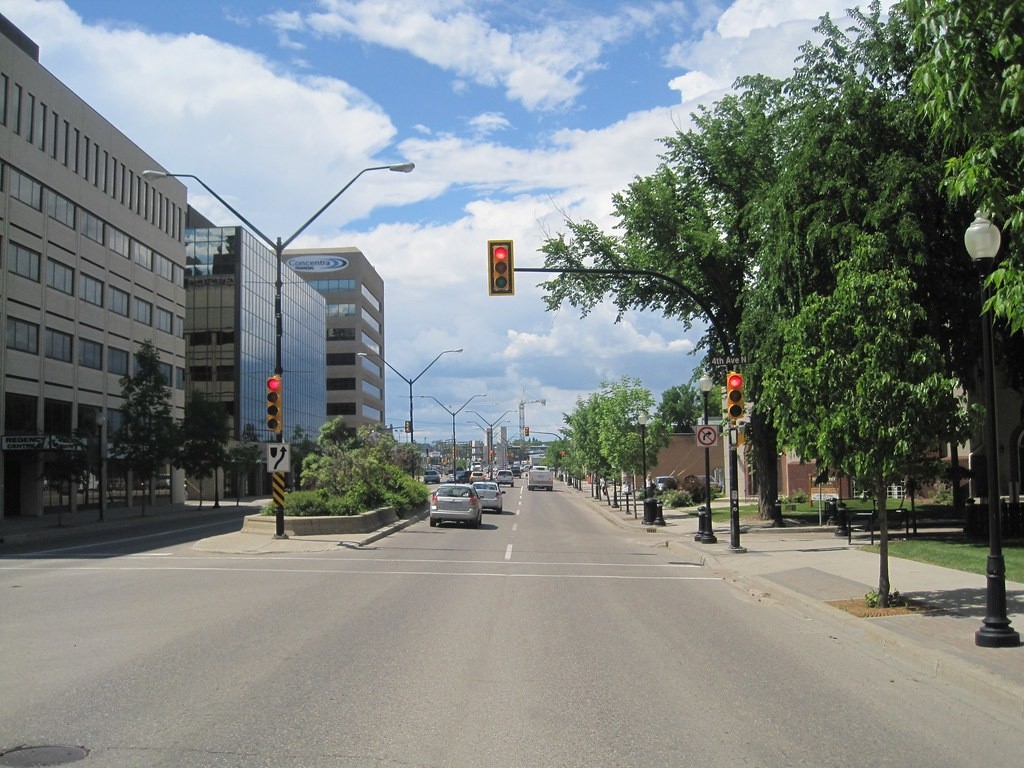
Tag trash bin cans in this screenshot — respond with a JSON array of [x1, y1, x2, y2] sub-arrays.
[[643, 498, 658, 526]]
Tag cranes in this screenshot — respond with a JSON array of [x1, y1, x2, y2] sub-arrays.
[[518, 399, 546, 471]]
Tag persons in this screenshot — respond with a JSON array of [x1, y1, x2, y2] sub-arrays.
[[647, 475, 655, 498], [600, 477, 609, 495]]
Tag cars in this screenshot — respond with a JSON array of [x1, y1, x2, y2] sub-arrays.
[[428, 482, 481, 530], [656, 476, 679, 492], [472, 482, 506, 514], [693, 475, 722, 494], [425, 460, 529, 489]]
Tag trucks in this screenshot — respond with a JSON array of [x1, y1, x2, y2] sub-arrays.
[[527, 464, 553, 491]]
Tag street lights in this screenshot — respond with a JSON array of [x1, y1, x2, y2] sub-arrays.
[[355, 349, 465, 507], [698, 375, 715, 533], [963, 207, 1022, 647], [418, 395, 488, 488], [639, 414, 649, 524], [139, 165, 428, 538], [465, 411, 518, 484]]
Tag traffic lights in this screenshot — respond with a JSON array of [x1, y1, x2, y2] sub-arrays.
[[726, 370, 745, 418], [525, 426, 530, 436], [487, 239, 515, 296]]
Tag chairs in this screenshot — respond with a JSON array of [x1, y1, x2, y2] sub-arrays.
[[448, 489, 457, 497], [476, 486, 481, 489], [489, 487, 494, 490]]
